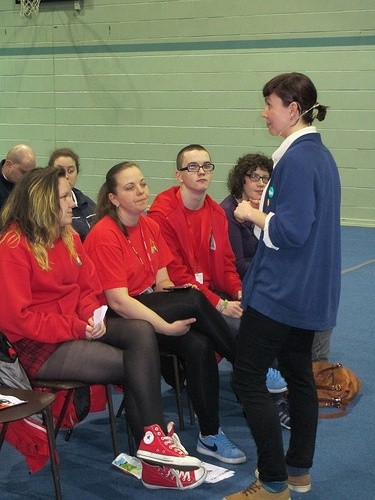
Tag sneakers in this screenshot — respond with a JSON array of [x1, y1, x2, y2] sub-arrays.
[[255, 468, 311, 492], [223, 480, 293, 500], [197, 426, 247, 464], [140, 459, 207, 490], [276, 400, 291, 431], [266, 368, 287, 393], [137, 421, 201, 471]]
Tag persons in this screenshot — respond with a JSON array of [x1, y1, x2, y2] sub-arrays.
[[0, 144, 36, 216], [84, 161, 286, 462], [220, 155, 315, 431], [222, 72, 341, 500], [146, 143, 289, 391], [0, 167, 210, 491], [49, 148, 99, 240]]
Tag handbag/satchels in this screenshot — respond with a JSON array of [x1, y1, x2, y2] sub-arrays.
[[312, 360, 359, 417]]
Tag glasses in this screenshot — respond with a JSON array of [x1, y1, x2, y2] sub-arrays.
[[246, 173, 269, 183], [179, 163, 214, 172]]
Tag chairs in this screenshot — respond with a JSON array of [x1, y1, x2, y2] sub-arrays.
[[0, 351, 194, 500]]
